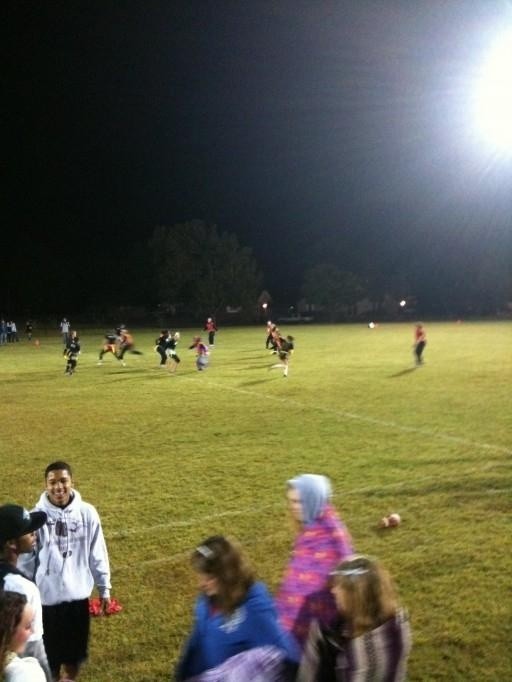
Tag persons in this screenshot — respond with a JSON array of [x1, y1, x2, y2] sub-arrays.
[[271, 327, 280, 354], [276, 337, 286, 352], [97, 330, 126, 367], [119, 329, 143, 359], [301, 555, 412, 682], [174, 536, 299, 681], [154, 330, 170, 367], [268, 335, 295, 378], [25, 319, 34, 341], [0, 503, 51, 678], [1, 591, 47, 681], [64, 331, 80, 375], [59, 317, 70, 342], [265, 321, 272, 348], [162, 332, 180, 373], [411, 322, 428, 366], [19, 461, 112, 682], [188, 336, 208, 371], [202, 317, 218, 347], [277, 472, 352, 635], [113, 324, 125, 341], [0, 319, 18, 343]]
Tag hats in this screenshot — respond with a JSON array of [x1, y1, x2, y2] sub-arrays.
[[0, 503, 48, 541]]
